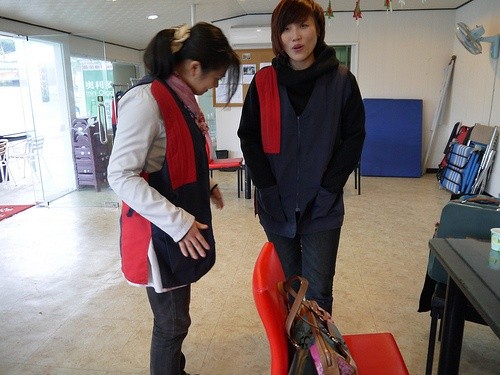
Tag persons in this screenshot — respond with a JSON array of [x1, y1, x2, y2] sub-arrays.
[[238, 0, 363, 314], [106, 20, 240, 375]]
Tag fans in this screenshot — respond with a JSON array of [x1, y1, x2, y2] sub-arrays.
[[455, 22, 500, 58]]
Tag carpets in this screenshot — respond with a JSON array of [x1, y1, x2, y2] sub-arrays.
[[0, 205, 34, 221]]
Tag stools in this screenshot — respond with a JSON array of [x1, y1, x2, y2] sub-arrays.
[[72, 116, 111, 192]]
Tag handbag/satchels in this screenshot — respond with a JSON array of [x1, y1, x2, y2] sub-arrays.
[[282, 275, 358, 375]]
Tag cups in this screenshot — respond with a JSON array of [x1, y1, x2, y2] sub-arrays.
[[490, 228, 500, 251]]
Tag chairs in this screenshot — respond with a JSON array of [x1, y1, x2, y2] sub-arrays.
[[0, 136, 44, 193], [418, 196, 500, 375], [435, 122, 500, 196], [253, 241, 410, 375]]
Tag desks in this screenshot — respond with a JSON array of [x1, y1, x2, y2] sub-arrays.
[[208, 158, 243, 199], [428, 238, 500, 375]]
